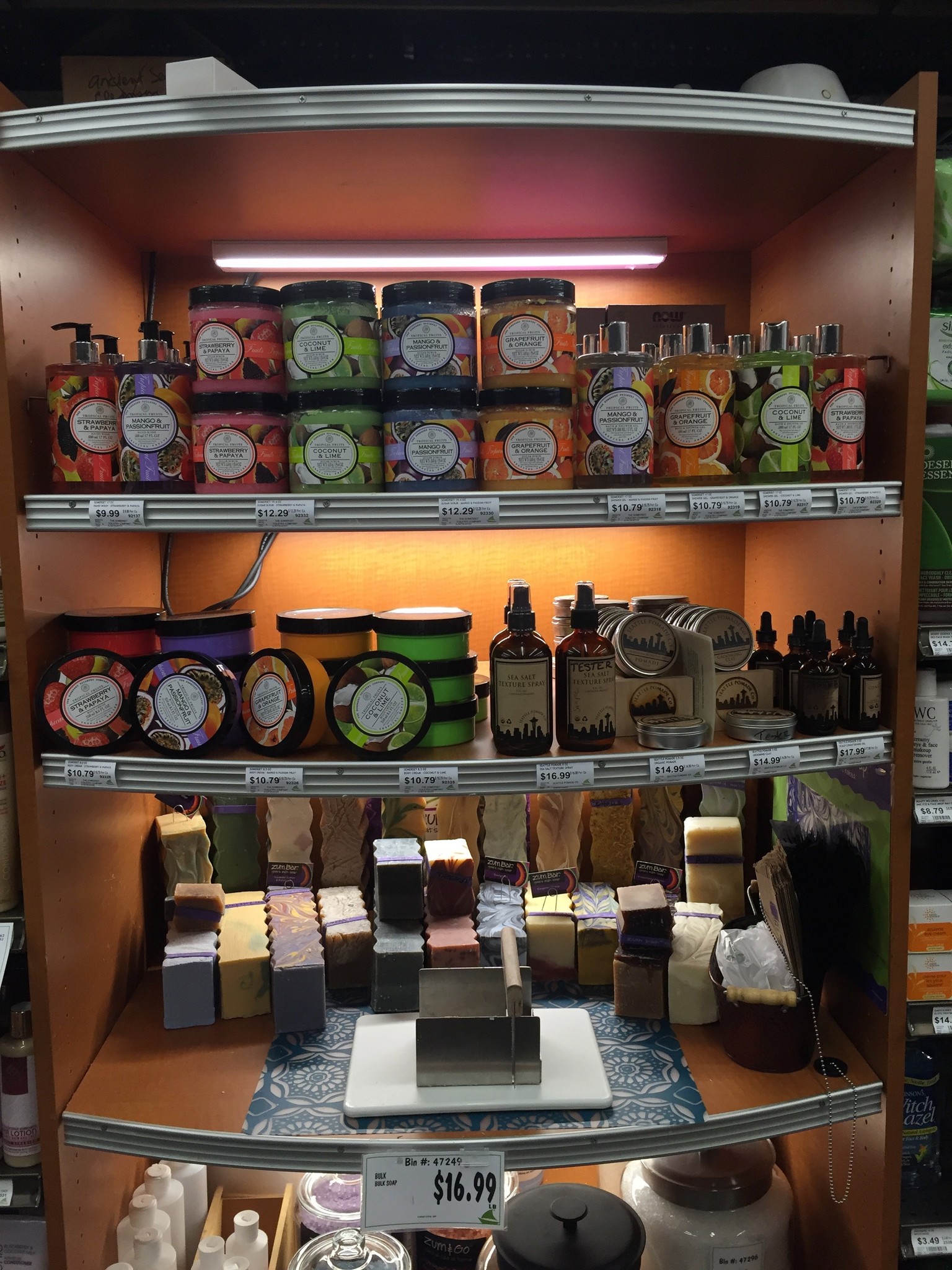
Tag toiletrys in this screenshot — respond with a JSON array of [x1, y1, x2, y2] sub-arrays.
[[199, 1208, 269, 1270], [2, 1002, 42, 1167], [44, 320, 194, 494], [572, 320, 868, 482], [104, 1158, 213, 1270], [489, 577, 623, 752]]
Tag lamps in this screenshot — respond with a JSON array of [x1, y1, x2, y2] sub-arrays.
[[210, 238, 667, 275]]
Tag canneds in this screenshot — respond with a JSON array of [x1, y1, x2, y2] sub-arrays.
[[481, 278, 577, 389], [191, 392, 289, 496], [380, 281, 480, 394], [383, 387, 479, 493], [478, 387, 575, 491], [285, 389, 386, 494], [187, 284, 287, 392], [280, 279, 382, 396]]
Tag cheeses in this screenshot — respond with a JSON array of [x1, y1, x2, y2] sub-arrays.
[[155, 784, 749, 1038]]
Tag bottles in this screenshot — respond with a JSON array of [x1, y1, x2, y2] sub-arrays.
[[190, 1210, 268, 1270], [829, 610, 883, 732], [555, 581, 616, 752], [912, 669, 952, 789], [642, 323, 737, 488], [728, 321, 868, 485], [104, 1194, 177, 1270], [488, 579, 552, 757], [133, 1159, 207, 1270], [573, 321, 656, 488], [747, 611, 783, 709], [783, 610, 840, 736], [901, 1033, 944, 1202], [0, 1002, 40, 1168]]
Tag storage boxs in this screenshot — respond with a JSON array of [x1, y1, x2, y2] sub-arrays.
[[908, 891, 951, 1000]]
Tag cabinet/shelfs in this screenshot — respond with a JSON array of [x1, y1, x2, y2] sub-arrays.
[[0, 81, 952, 1270]]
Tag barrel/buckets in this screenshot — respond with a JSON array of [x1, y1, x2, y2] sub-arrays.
[[708, 915, 821, 1073]]
